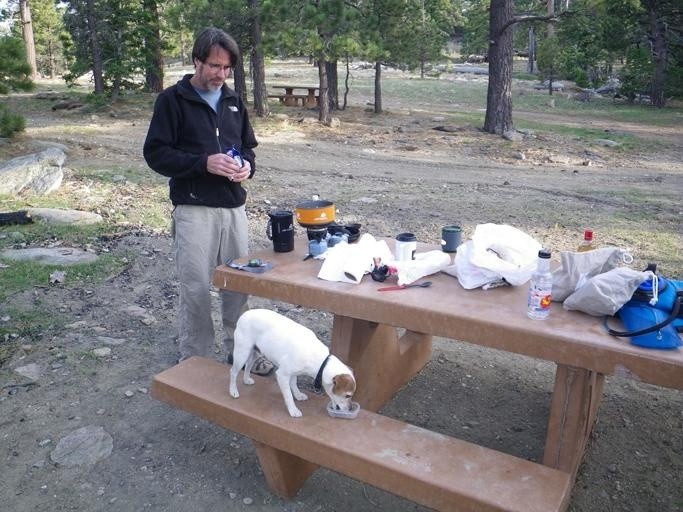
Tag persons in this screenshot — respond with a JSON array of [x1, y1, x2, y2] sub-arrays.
[[144, 28, 276, 374]]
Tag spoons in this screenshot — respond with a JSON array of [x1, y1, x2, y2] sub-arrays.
[[376, 280, 432, 293]]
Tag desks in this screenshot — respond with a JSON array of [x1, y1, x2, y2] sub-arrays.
[[213, 234, 683, 498], [272, 86, 320, 110]]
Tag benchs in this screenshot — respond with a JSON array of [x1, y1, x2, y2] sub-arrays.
[[148, 356, 573, 512], [267, 95, 320, 110]]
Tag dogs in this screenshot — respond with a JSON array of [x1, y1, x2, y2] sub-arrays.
[[229, 308, 357, 418]]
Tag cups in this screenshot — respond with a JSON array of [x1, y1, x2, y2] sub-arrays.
[[394, 232, 418, 262]]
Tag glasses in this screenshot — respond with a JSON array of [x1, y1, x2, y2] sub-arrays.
[[203, 60, 235, 75]]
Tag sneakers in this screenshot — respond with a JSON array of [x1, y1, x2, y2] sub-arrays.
[[227, 352, 274, 377]]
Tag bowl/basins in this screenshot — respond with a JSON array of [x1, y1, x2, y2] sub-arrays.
[[325, 400, 361, 420]]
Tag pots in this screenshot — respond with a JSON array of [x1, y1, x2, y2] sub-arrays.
[[295, 193, 336, 230]]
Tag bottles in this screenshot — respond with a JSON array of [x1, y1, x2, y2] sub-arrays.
[[265, 208, 296, 253], [526, 248, 556, 322], [577, 230, 597, 253], [442, 224, 463, 253]]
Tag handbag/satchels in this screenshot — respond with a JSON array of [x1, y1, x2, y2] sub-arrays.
[[551, 246, 682, 350]]
